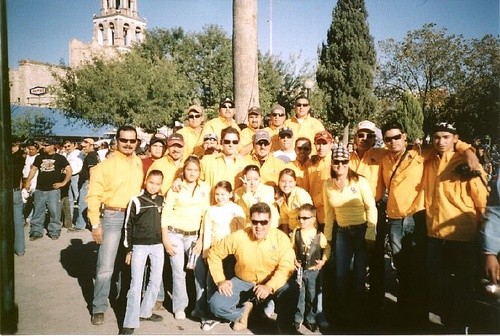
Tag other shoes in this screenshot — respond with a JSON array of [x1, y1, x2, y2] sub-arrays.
[[92, 300, 329, 335], [28, 227, 85, 240]]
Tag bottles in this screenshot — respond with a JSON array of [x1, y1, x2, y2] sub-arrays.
[[186, 241, 197, 269]]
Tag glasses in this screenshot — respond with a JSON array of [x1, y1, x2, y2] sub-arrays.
[[118, 138, 136, 143], [359, 134, 372, 138], [222, 104, 233, 108], [334, 160, 348, 164], [252, 220, 268, 225], [297, 216, 312, 220], [189, 115, 200, 118], [385, 134, 401, 142], [272, 101, 309, 118], [204, 137, 239, 145]]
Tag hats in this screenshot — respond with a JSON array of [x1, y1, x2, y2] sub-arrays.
[[314, 131, 332, 144], [42, 140, 56, 145], [248, 107, 260, 114], [279, 127, 292, 135], [220, 97, 233, 107], [357, 121, 377, 133], [255, 130, 270, 143], [150, 133, 167, 145], [80, 138, 94, 146], [432, 122, 456, 135], [187, 105, 201, 113], [168, 133, 184, 147]]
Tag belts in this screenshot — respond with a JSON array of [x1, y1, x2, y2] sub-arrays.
[[168, 226, 196, 235], [72, 173, 79, 176], [105, 206, 127, 211]]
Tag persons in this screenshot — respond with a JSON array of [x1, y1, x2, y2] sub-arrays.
[[173, 126, 251, 194], [239, 96, 423, 332], [11, 136, 72, 255], [160, 156, 211, 321], [174, 105, 204, 159], [202, 181, 247, 331], [380, 120, 482, 325], [482, 165, 500, 285], [204, 97, 243, 139], [86, 126, 144, 326], [69, 138, 100, 233], [117, 170, 165, 335], [143, 133, 186, 196], [142, 133, 167, 176], [234, 166, 282, 229], [98, 139, 117, 161], [62, 138, 85, 222], [25, 139, 73, 240], [207, 203, 301, 335], [424, 119, 489, 327]]
